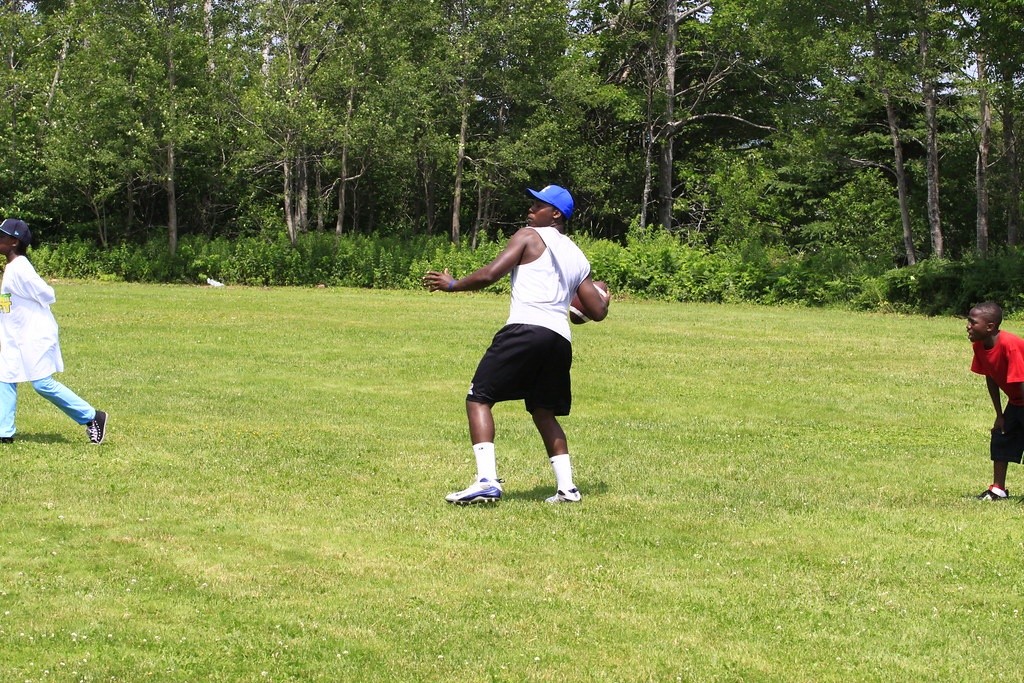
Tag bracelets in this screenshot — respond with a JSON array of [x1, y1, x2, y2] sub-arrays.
[[447, 279, 457, 292]]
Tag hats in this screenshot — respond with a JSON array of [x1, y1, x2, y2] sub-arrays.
[[0, 218, 31, 246], [524, 184, 574, 220]]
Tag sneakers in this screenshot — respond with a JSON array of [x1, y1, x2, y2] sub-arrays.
[[544, 486, 581, 504], [977, 485, 1009, 501], [86, 410, 108, 446], [444, 478, 502, 505]]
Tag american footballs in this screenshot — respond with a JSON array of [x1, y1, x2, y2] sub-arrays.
[[570, 282, 607, 324]]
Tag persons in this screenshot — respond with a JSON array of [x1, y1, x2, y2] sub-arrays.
[[422, 183, 612, 507], [964, 301, 1024, 502], [0, 217, 108, 446]]
